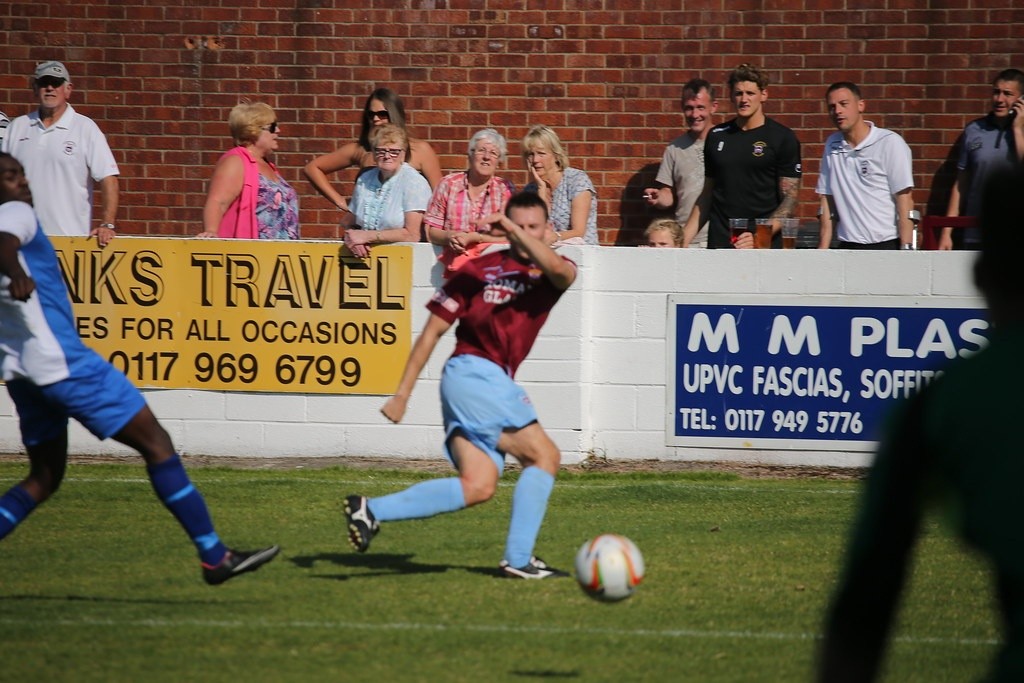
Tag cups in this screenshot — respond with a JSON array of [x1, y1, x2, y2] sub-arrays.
[[728, 219, 750, 249], [778, 218, 800, 249], [755, 219, 774, 249]]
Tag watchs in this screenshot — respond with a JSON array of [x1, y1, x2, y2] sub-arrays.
[[99, 223, 116, 229]]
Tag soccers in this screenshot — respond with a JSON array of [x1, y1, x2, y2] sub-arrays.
[[576, 533, 645, 602]]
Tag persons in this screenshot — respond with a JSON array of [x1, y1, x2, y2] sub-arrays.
[[520, 123, 599, 247], [195, 102, 300, 239], [0, 61, 120, 248], [682, 63, 802, 250], [814, 82, 915, 250], [643, 219, 684, 248], [344, 123, 432, 260], [304, 87, 442, 214], [939, 69, 1024, 250], [645, 78, 719, 248], [811, 159, 1024, 683], [423, 128, 517, 256], [343, 192, 577, 582], [0, 152, 279, 584]]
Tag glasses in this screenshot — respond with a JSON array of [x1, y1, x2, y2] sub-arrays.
[[35, 78, 66, 89], [374, 146, 407, 158], [366, 109, 389, 120], [258, 122, 277, 134], [472, 147, 501, 159]]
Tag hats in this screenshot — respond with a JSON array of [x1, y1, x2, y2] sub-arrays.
[[31, 61, 70, 84]]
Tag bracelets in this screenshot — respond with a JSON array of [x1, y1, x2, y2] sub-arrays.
[[376, 231, 380, 241], [554, 232, 561, 242], [900, 244, 913, 249], [479, 234, 483, 243]]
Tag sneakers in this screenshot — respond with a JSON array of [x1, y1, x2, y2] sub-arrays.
[[200, 545, 281, 586], [342, 494, 380, 550], [497, 555, 571, 584]]
